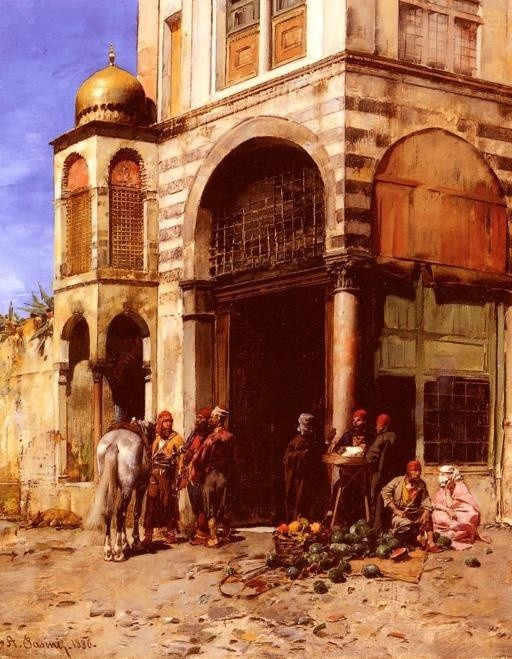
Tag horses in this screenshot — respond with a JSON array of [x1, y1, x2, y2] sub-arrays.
[[81, 419, 159, 563]]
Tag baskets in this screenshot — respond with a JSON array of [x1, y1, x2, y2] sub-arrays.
[[270, 532, 321, 568]]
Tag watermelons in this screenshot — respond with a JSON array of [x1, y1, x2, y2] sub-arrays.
[[432, 532, 452, 547], [266, 520, 409, 593]]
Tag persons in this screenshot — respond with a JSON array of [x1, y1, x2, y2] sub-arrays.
[[133, 403, 494, 553]]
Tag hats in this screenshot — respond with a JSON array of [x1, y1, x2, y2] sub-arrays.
[[406, 460, 422, 471], [376, 414, 391, 426], [298, 413, 315, 425], [212, 404, 230, 418], [198, 407, 213, 419], [352, 409, 369, 420], [157, 411, 173, 422], [440, 465, 454, 473]]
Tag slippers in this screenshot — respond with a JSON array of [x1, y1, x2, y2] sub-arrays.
[[140, 534, 232, 549], [421, 542, 443, 554]]
